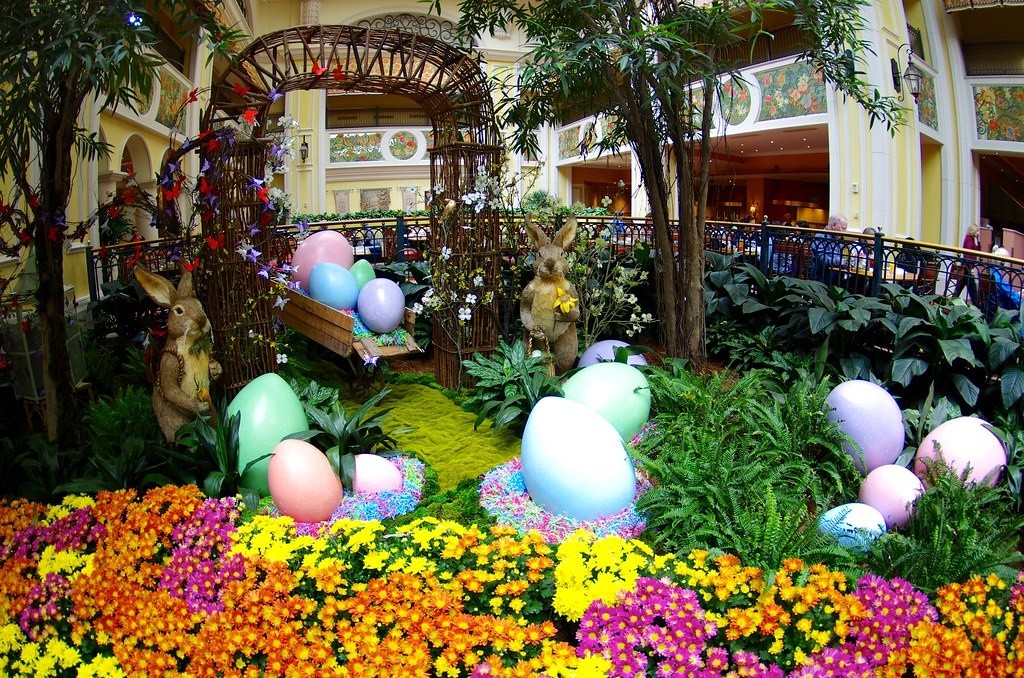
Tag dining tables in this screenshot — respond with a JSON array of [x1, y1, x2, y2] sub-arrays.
[[406, 233, 434, 248], [830, 266, 942, 295], [706, 242, 760, 269]]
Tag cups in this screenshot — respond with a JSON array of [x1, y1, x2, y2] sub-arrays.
[[890, 264, 895, 274]]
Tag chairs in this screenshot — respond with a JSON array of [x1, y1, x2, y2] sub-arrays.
[[586, 224, 1024, 325]]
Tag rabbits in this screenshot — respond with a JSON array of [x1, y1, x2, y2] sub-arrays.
[[132, 261, 224, 444], [519, 210, 582, 386]]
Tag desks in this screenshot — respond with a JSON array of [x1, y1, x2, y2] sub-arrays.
[[352, 245, 371, 256]]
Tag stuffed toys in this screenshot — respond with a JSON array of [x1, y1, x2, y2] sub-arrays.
[[520, 212, 581, 376], [134, 259, 221, 448]]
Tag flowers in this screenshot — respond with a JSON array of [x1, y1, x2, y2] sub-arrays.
[[409, 159, 651, 354], [0, 485, 1024, 678], [237, 112, 302, 224], [276, 353, 289, 365]]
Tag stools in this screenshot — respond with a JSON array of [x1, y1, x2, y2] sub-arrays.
[[24, 382, 94, 434]]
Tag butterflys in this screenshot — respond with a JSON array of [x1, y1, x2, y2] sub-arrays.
[[362, 346, 384, 369], [0, 59, 351, 333]]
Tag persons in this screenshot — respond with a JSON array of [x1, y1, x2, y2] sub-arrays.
[[952, 223, 981, 309], [602, 211, 653, 241], [988, 247, 1024, 311], [734, 216, 919, 284]]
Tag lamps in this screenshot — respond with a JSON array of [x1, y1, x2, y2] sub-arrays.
[[300, 135, 309, 163]]
